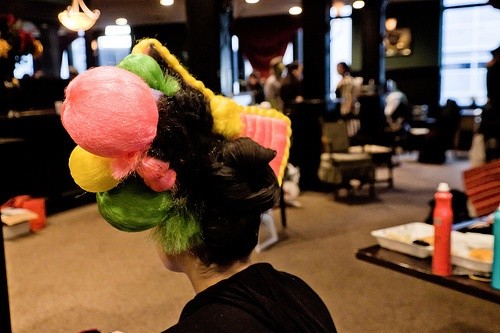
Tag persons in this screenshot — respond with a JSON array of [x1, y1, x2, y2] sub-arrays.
[[248, 47, 500, 190], [60, 39, 338, 333]]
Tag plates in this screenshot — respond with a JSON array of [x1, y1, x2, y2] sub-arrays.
[[451, 232, 494, 272], [371, 222, 461, 258]]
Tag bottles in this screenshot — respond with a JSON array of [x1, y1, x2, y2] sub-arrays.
[[433, 183, 452, 276], [491, 204, 500, 290]]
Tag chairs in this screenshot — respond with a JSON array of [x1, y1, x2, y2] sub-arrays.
[[318, 119, 393, 205]]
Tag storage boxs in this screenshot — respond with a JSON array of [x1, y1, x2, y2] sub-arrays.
[[1, 207, 39, 239]]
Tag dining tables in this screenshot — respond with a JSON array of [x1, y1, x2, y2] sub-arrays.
[[357, 212, 500, 304]]
